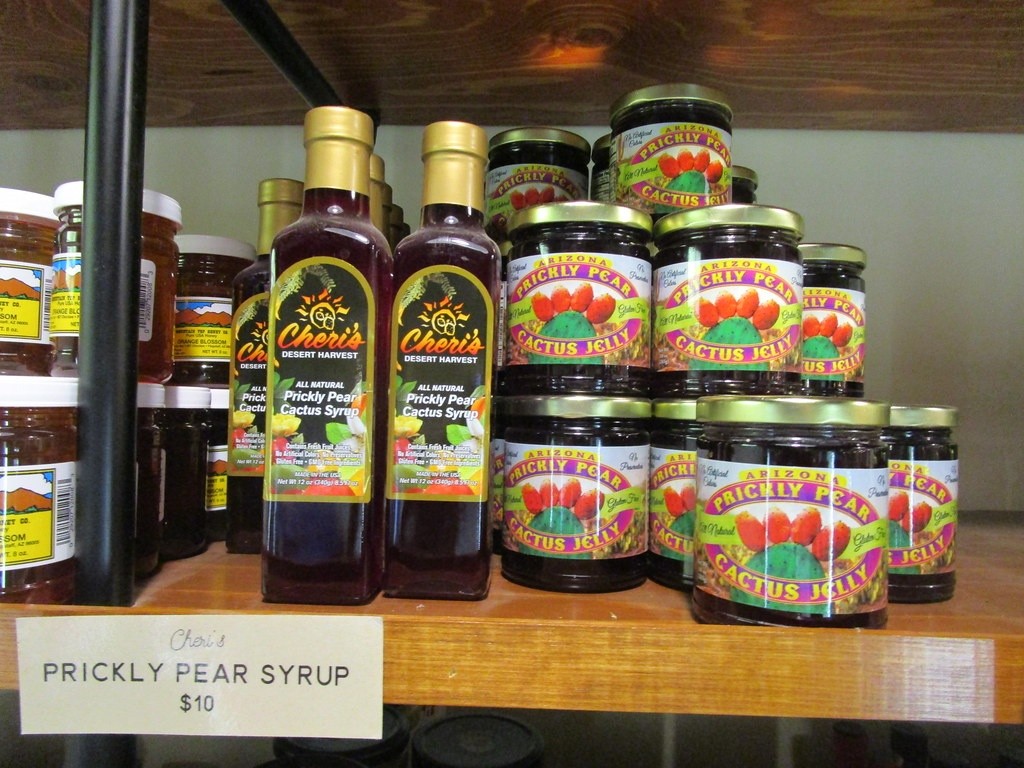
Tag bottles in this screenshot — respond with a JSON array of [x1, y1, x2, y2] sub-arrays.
[[226, 105, 501, 604]]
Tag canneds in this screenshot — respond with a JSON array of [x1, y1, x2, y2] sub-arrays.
[[0, 178, 260, 606], [484, 83, 959, 631]]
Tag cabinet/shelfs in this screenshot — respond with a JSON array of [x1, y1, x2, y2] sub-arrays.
[[0, 0, 1024, 725]]
